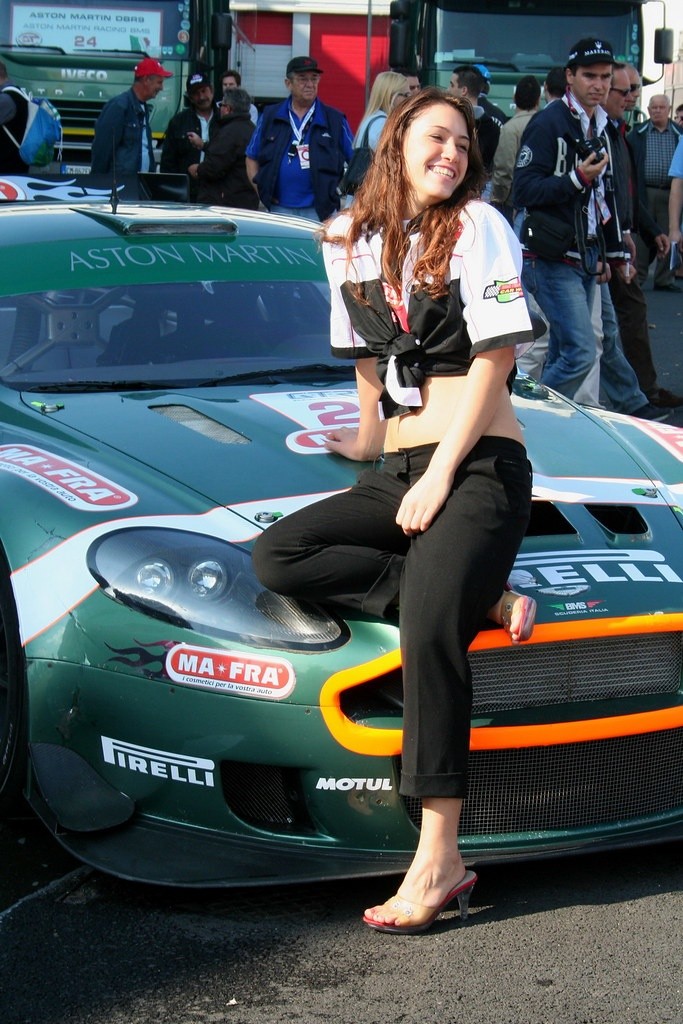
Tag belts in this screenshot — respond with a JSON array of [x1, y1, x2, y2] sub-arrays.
[[646, 183, 671, 190]]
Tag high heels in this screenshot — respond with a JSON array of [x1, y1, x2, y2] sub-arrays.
[[500, 579, 537, 641], [363, 870, 478, 934]]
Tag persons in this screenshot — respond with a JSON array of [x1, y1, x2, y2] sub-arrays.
[[448, 36, 683, 430], [214, 70, 258, 126], [244, 56, 355, 224], [90, 57, 174, 182], [0, 62, 30, 176], [160, 74, 220, 202], [187, 89, 259, 211], [345, 68, 421, 213], [248, 89, 537, 936]]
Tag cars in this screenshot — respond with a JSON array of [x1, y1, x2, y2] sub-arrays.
[[0, 172, 683, 890]]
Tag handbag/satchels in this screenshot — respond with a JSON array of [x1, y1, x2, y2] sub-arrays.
[[336, 115, 388, 200]]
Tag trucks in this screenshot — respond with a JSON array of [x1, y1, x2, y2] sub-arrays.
[[0, 0, 233, 173], [385, 0, 674, 137]]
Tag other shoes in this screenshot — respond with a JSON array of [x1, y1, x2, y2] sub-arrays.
[[644, 388, 683, 408], [653, 281, 683, 293], [630, 403, 673, 421]]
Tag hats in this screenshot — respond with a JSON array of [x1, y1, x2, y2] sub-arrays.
[[135, 58, 173, 78], [286, 56, 323, 75], [187, 72, 210, 96], [569, 37, 625, 66]]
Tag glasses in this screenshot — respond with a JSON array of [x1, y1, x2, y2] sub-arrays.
[[218, 103, 226, 107], [610, 88, 631, 96], [630, 83, 641, 92], [398, 92, 411, 97], [680, 116, 683, 121], [291, 76, 320, 84]]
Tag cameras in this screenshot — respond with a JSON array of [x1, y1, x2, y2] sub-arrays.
[[575, 137, 605, 165]]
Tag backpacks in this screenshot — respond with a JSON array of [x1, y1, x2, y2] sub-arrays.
[[0, 86, 63, 167]]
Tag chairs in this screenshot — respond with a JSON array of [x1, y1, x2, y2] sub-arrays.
[[120, 294, 199, 365]]
